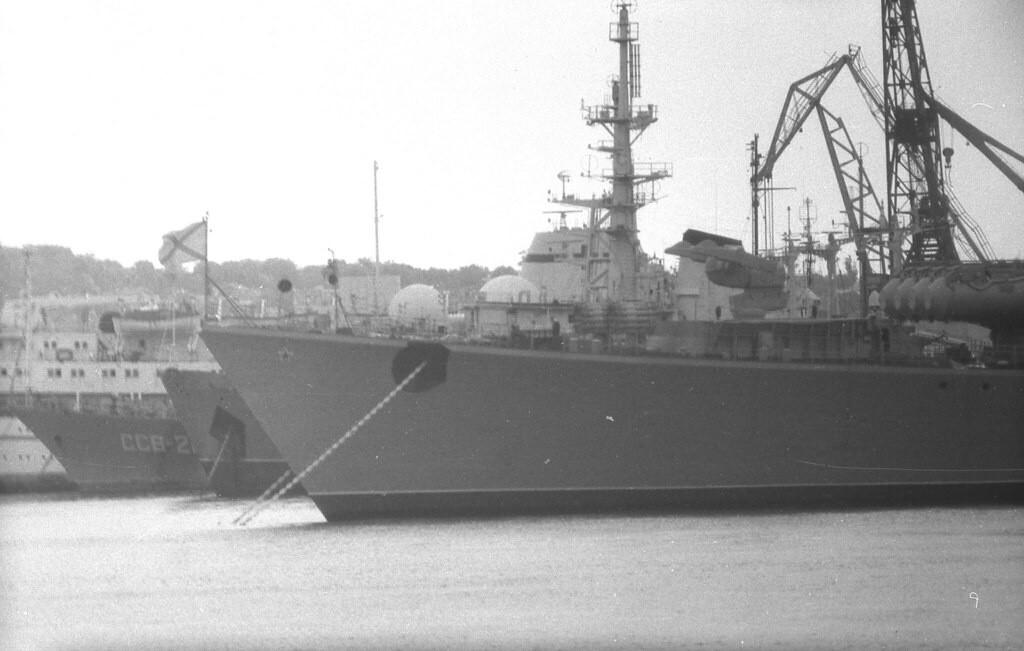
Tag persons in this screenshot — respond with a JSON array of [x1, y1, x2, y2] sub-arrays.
[[512, 324, 527, 347], [550, 316, 560, 350], [960, 344, 976, 365], [715, 304, 721, 320]]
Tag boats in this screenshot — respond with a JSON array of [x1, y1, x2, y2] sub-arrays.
[[149, 0, 679, 494], [7, 398, 206, 494], [0, 248, 356, 493], [200, 0, 1024, 521]]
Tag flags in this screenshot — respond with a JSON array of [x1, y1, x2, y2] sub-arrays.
[[160, 223, 205, 267]]
